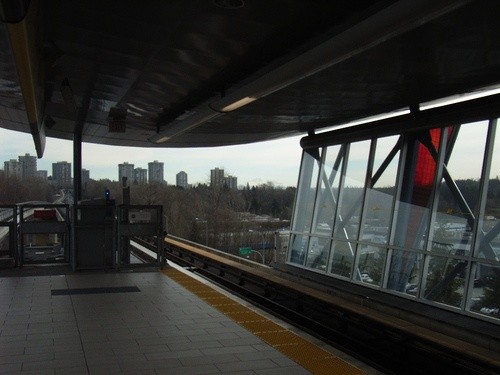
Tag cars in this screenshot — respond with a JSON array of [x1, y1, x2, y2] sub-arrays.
[[361, 273, 418, 295], [471, 297, 500, 318]]
